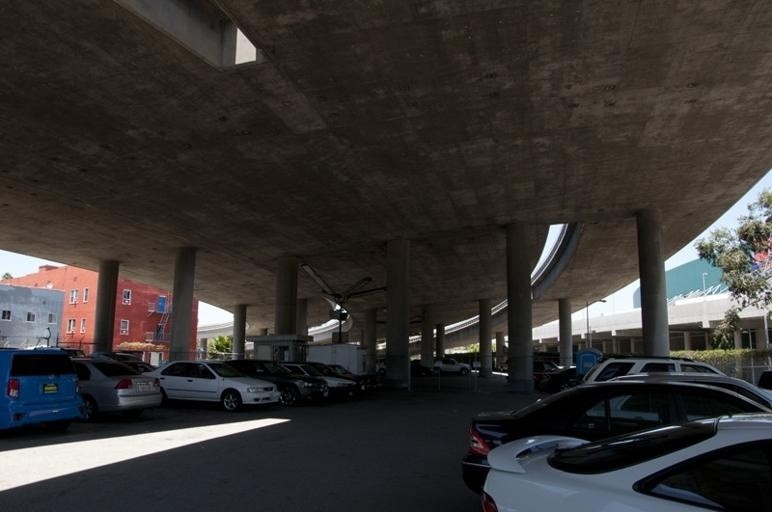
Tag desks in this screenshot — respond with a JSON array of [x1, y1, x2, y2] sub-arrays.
[[701, 271, 709, 296], [585, 299, 607, 350]]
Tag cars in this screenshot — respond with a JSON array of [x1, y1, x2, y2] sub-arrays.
[[0, 346, 382, 432], [480, 413, 772, 512], [456, 352, 771, 422], [460, 373, 772, 495]]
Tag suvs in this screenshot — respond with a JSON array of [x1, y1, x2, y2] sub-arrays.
[[433, 355, 471, 375]]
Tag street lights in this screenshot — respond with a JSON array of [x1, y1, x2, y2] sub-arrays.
[[701, 271, 709, 296], [585, 299, 607, 350]]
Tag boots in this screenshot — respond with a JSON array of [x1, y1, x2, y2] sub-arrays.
[[433, 355, 471, 375]]
